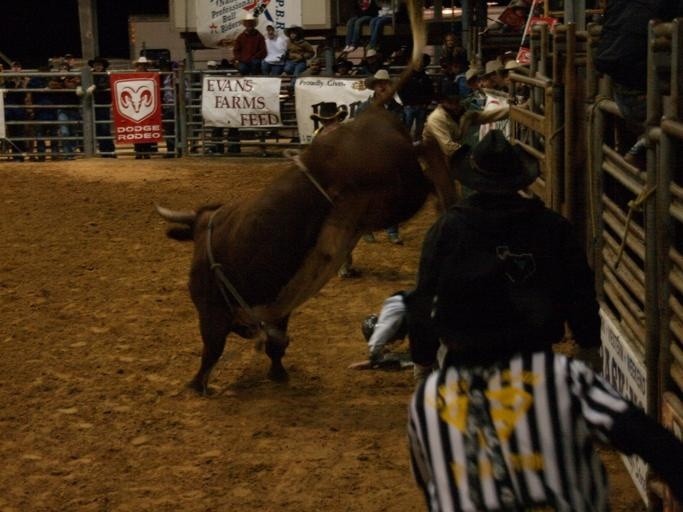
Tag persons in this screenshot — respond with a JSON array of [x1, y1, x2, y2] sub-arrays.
[[358, 33, 523, 245], [407, 270, 683, 512], [0, 55, 242, 157], [343, 0, 398, 57], [231, 13, 315, 78], [411, 130, 604, 389], [348, 290, 418, 369]]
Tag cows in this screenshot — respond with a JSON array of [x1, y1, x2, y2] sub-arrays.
[[151, 0, 454, 395]]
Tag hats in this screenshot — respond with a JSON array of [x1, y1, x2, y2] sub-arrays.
[[283, 24, 304, 37], [365, 69, 392, 90], [465, 60, 521, 88], [239, 14, 259, 26], [88, 56, 110, 69], [131, 57, 152, 66], [332, 57, 353, 73], [448, 129, 541, 194]]
